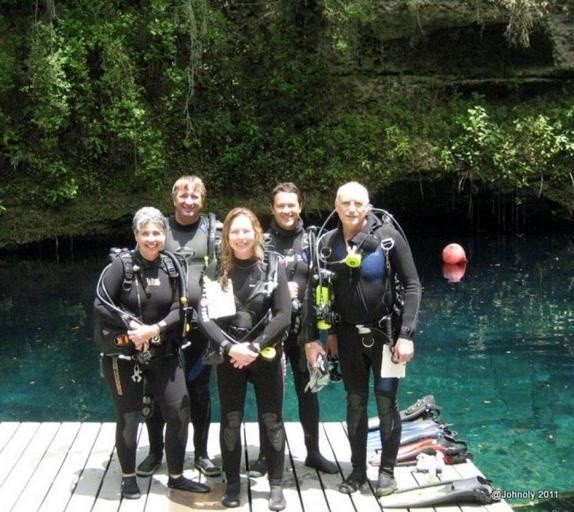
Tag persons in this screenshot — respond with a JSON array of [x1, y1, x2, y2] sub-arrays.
[[92, 207, 210, 499], [247, 184, 338, 479], [135, 177, 220, 478], [303, 181, 422, 495], [198, 206, 291, 508]]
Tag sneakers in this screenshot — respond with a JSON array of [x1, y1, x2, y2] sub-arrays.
[[269, 483, 286, 510], [166, 475, 212, 494], [223, 493, 240, 509], [340, 470, 367, 493], [376, 467, 397, 496], [249, 451, 268, 478], [304, 453, 339, 475], [194, 455, 224, 478], [137, 455, 163, 477], [120, 475, 142, 500]]
